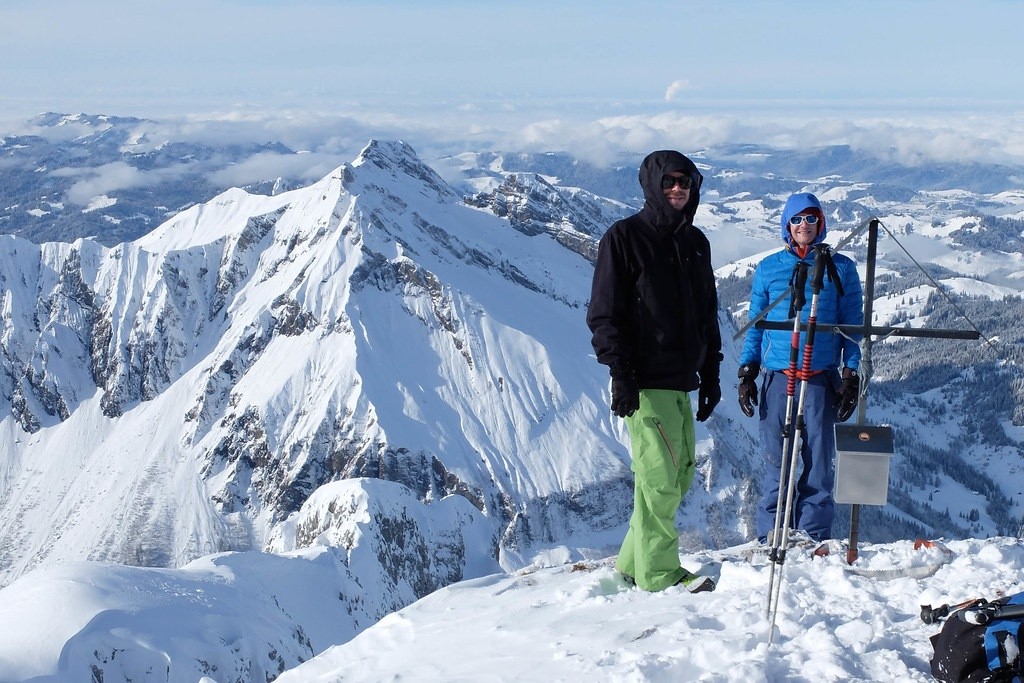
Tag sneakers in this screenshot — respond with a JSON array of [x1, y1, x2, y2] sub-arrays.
[[620, 571, 637, 585], [674, 572, 715, 593]]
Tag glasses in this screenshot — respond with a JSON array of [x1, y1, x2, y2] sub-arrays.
[[662, 175, 693, 189], [788, 215, 819, 226]]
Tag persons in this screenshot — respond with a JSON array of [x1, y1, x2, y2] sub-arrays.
[[738, 193, 863, 556], [585, 150, 724, 593]]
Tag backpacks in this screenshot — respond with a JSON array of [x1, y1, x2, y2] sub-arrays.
[[930, 592, 1024, 683]]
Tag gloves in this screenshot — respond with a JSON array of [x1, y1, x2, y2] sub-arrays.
[[696, 370, 722, 422], [738, 364, 759, 418], [611, 372, 640, 417], [832, 367, 859, 423]]
